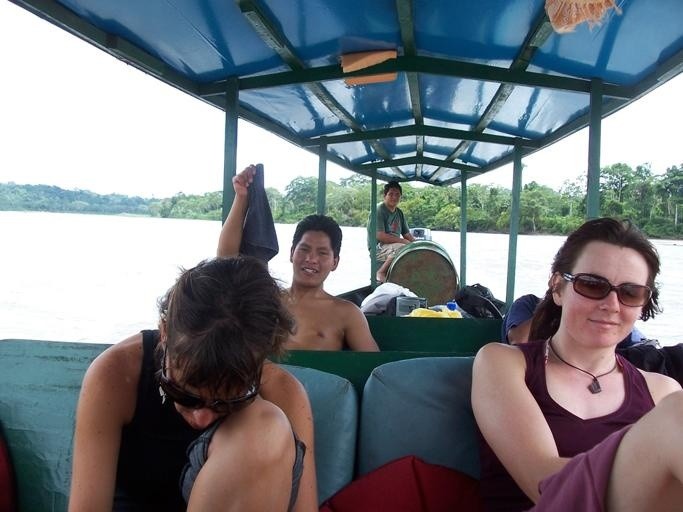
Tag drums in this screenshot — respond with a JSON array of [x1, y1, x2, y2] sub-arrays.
[[385, 240, 461, 307]]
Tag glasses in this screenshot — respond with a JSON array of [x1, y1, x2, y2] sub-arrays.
[[386, 192, 400, 197], [561, 273, 653, 307], [160, 341, 263, 410]]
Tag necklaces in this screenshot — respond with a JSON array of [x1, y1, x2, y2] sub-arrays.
[[548, 336, 618, 394]]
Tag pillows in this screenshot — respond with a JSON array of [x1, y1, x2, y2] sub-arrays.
[[266, 361, 356, 505], [358, 356, 502, 480]]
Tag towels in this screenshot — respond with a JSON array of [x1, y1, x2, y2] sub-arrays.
[[240, 163, 278, 263]]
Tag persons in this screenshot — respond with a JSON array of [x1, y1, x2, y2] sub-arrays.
[[216, 163, 381, 352], [367, 180, 416, 283], [502, 294, 645, 357], [469, 216, 683, 512], [66, 255, 320, 512]]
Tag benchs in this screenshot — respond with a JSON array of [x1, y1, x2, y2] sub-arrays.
[[360, 314, 505, 354], [2, 338, 479, 512]]
